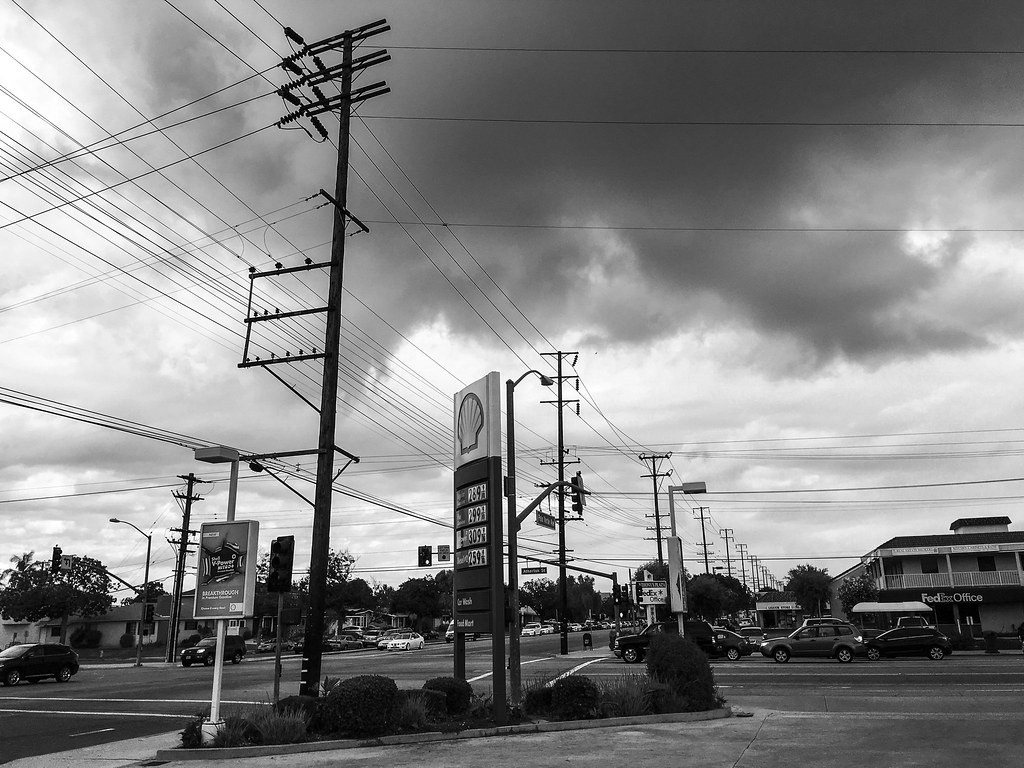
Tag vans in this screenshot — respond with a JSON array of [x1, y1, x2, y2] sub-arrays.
[[445, 619, 474, 643]]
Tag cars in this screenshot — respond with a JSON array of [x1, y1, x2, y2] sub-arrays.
[[376, 628, 413, 645], [258, 637, 297, 653], [377, 633, 400, 651], [294, 637, 305, 654], [713, 629, 752, 661], [387, 633, 425, 652], [328, 635, 363, 651], [760, 624, 865, 664], [522, 623, 542, 637], [739, 627, 767, 651], [570, 620, 639, 632], [864, 627, 953, 661], [361, 630, 385, 648], [420, 630, 440, 640], [542, 625, 554, 635]]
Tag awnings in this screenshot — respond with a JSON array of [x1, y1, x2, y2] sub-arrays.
[[851, 601, 933, 613]]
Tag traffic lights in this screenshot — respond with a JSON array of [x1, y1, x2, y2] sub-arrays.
[[636, 585, 643, 604], [52, 547, 63, 572], [571, 477, 583, 515], [146, 604, 155, 623], [612, 585, 621, 604], [268, 536, 294, 591], [622, 584, 628, 598]]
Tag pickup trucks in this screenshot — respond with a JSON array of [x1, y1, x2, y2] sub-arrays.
[[896, 616, 936, 629]]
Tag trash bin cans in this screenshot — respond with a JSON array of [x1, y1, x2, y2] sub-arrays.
[[980, 630, 1000, 654]]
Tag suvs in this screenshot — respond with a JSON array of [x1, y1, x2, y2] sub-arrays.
[[342, 625, 365, 638], [180, 635, 247, 667], [800, 618, 850, 635], [715, 619, 737, 632], [613, 621, 718, 663], [739, 618, 755, 629], [0, 643, 80, 686]]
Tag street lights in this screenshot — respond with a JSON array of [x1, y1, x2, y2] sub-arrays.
[[110, 518, 151, 665], [668, 481, 706, 638], [713, 567, 723, 575], [506, 371, 555, 698], [195, 446, 240, 746]]
